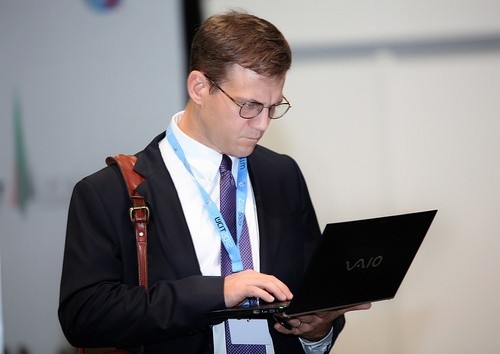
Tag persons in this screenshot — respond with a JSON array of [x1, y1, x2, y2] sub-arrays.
[[58, 13, 372, 354]]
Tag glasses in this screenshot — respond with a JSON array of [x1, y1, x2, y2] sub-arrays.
[[204, 73, 291, 119]]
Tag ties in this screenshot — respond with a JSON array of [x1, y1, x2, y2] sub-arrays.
[[219, 154, 267, 354]]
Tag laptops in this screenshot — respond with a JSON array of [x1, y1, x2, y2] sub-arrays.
[[207, 209, 438, 319]]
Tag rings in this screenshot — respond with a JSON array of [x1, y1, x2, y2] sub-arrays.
[[296, 321, 304, 328]]
